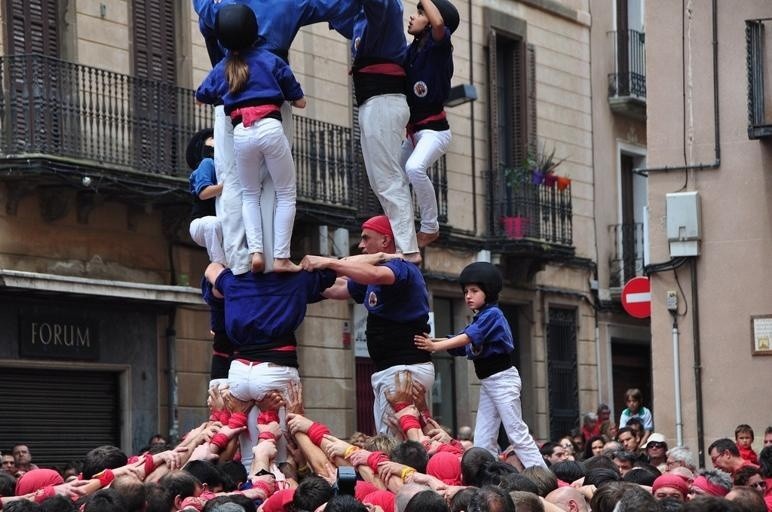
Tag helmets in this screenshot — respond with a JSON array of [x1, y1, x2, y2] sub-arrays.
[[418, 0, 460, 35], [215, 3, 257, 46], [458, 262, 503, 294], [186, 128, 214, 169]]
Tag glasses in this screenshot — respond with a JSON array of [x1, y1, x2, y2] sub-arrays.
[[750, 481, 766, 489], [648, 442, 665, 448]]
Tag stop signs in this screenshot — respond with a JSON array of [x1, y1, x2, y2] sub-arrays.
[[619, 276, 650, 319]]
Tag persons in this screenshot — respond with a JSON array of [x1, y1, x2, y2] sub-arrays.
[[199, 257, 236, 381], [333, 1, 424, 267], [300, 213, 436, 442], [202, 252, 408, 470], [192, 1, 308, 273], [183, 126, 225, 267], [403, 1, 461, 253], [189, 1, 331, 276], [0, 370, 771, 512], [412, 260, 548, 471]]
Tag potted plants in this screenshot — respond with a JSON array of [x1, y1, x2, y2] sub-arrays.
[[498, 162, 531, 240]]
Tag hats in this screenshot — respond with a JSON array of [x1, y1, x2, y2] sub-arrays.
[[362, 216, 392, 236], [640, 433, 668, 451]]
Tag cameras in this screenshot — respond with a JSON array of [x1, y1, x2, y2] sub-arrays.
[[332, 466, 357, 497]]
